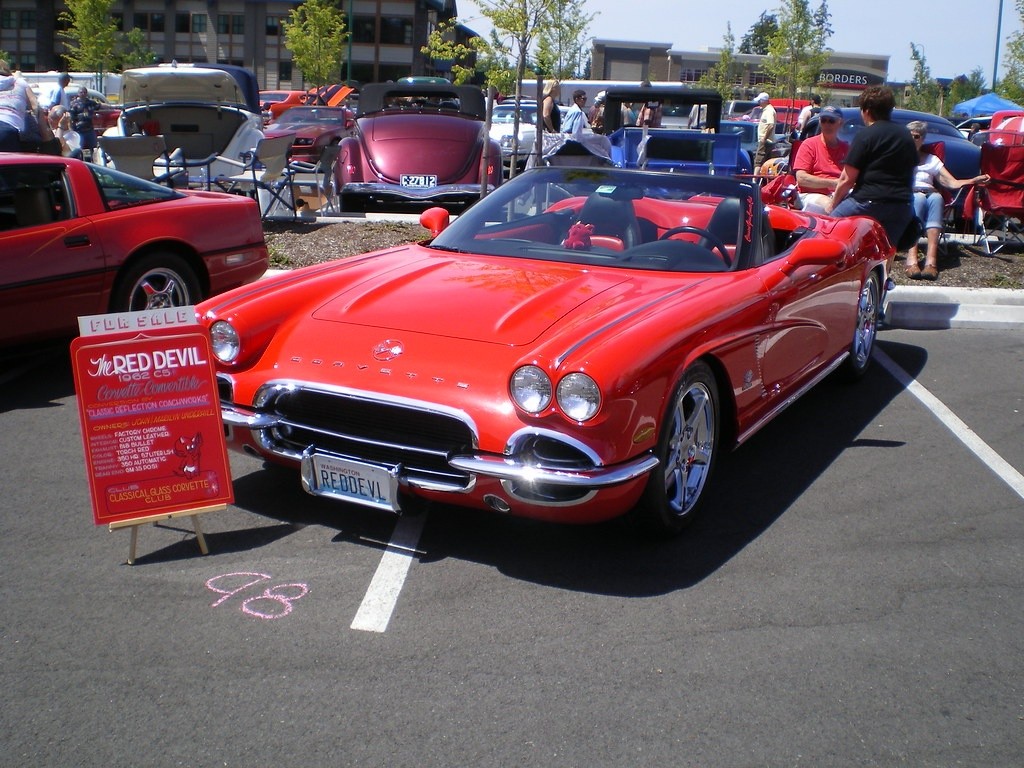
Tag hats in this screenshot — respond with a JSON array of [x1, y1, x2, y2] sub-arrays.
[[59, 72, 72, 81], [595, 91, 607, 102], [819, 106, 842, 121], [753, 91, 770, 102]]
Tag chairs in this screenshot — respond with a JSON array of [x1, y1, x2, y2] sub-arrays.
[[13, 186, 50, 227], [785, 139, 1024, 256], [576, 188, 641, 252], [439, 101, 458, 109], [69, 134, 342, 222], [699, 197, 773, 270]]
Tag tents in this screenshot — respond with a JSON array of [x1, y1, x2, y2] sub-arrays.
[[953, 93, 1024, 118]]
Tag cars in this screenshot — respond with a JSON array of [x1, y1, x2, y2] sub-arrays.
[[398, 76, 451, 84], [88, 109, 123, 140], [488, 105, 570, 172], [796, 106, 981, 223], [975, 110, 1024, 148], [93, 66, 265, 192], [693, 120, 789, 167], [721, 100, 760, 120], [258, 85, 355, 125], [0, 151, 269, 340], [28, 83, 114, 111], [264, 105, 354, 171], [956, 116, 992, 140], [499, 100, 539, 105]]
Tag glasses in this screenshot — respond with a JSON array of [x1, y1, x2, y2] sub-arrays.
[[578, 96, 587, 101], [821, 119, 838, 124], [912, 135, 922, 140]]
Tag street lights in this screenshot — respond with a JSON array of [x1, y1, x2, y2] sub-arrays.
[[910, 44, 925, 93]]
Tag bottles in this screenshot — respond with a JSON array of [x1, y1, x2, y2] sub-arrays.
[[199, 167, 206, 182]]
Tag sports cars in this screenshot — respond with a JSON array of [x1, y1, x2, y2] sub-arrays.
[[194, 166, 898, 535], [336, 82, 504, 214]]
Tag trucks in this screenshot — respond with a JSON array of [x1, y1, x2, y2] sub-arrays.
[[18, 72, 121, 98]]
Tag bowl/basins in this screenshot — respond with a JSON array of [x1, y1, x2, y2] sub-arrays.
[[500, 133, 513, 146], [522, 131, 535, 140]]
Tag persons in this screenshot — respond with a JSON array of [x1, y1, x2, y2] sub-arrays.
[[687, 104, 708, 128], [563, 89, 587, 134], [541, 79, 561, 134], [793, 94, 992, 279], [0, 59, 110, 161], [751, 92, 777, 184], [392, 95, 442, 108], [588, 79, 664, 133]]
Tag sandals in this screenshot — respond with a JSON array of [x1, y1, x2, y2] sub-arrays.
[[921, 263, 939, 280], [905, 263, 920, 278]]
